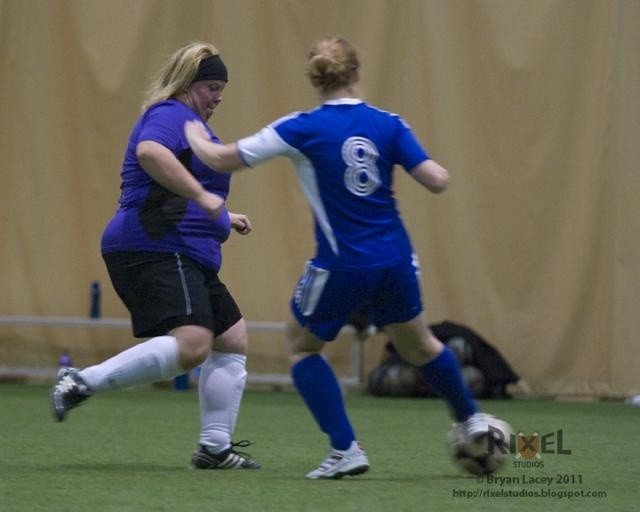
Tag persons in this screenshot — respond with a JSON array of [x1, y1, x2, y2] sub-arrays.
[[184, 36, 489, 479], [51, 42, 263, 472]]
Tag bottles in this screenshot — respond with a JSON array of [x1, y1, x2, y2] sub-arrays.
[[59, 348, 69, 368], [88, 280, 102, 318]]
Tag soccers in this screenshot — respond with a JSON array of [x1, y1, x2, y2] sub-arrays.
[[446, 411, 512, 473]]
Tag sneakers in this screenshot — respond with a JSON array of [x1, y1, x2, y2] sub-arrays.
[[191, 440, 260, 469], [305, 440, 369, 478], [468, 415, 513, 448], [54, 368, 91, 421]]
